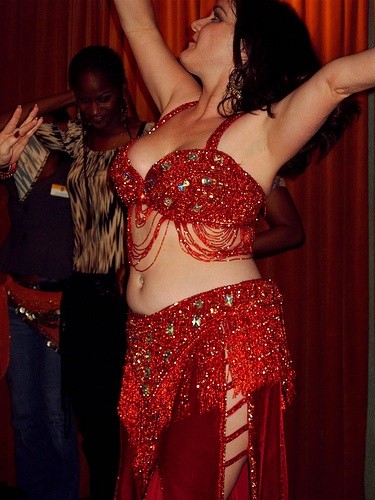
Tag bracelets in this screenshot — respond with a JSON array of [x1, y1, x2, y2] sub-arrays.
[[0, 162, 18, 179]]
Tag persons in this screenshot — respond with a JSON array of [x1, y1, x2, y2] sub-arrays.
[[0, 44, 306, 500], [106, 0, 375, 500]]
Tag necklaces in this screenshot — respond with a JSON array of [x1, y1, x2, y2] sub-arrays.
[[92, 121, 124, 152]]
